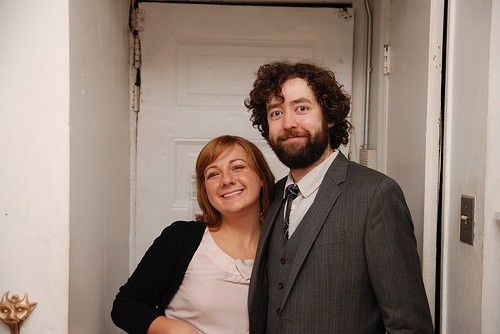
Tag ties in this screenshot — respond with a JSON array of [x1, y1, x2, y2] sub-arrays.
[[283, 184, 299, 247]]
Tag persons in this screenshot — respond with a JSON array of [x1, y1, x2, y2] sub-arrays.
[[244, 59, 433, 334], [110, 134, 277, 334]]
